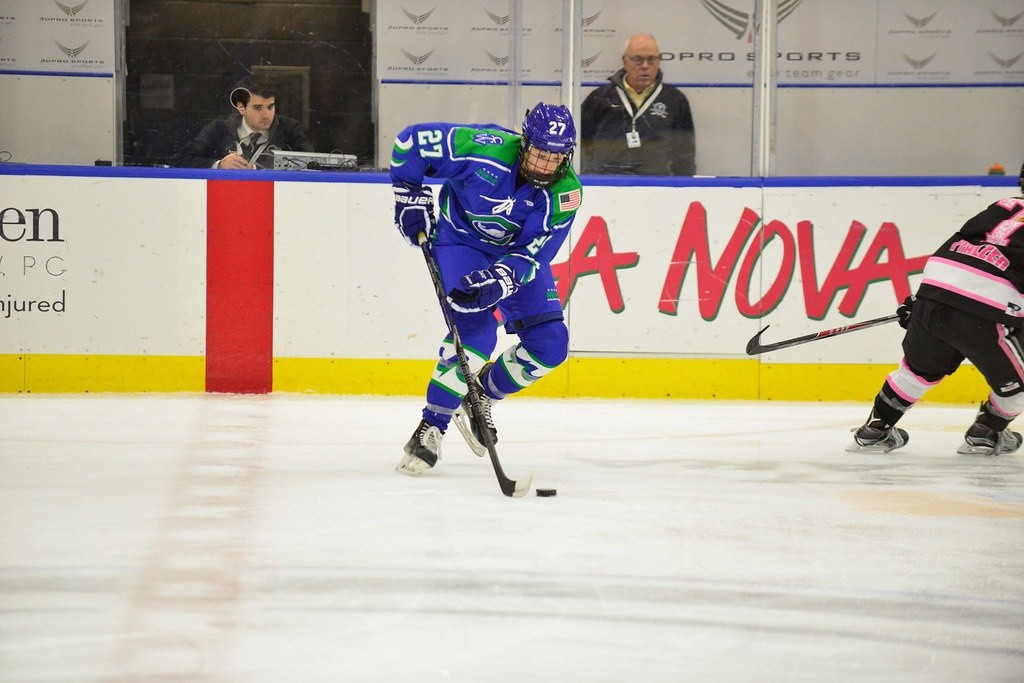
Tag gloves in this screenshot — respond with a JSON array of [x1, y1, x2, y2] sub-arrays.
[[444, 264, 516, 318], [897, 296, 920, 330], [389, 180, 436, 247]]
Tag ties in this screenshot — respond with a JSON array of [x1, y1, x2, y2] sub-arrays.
[[245, 130, 262, 155]]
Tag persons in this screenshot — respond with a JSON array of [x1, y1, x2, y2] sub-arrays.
[[170, 79, 316, 170], [581, 34, 696, 178], [391, 105, 581, 476], [844, 165, 1024, 456]]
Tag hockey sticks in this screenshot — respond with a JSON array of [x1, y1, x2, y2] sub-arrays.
[[745, 311, 911, 356], [414, 230, 534, 497]]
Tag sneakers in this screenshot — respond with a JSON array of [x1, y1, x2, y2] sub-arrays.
[[845, 418, 910, 457], [958, 421, 1024, 459], [396, 413, 446, 477], [447, 362, 501, 456]]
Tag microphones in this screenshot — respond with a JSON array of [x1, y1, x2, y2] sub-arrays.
[[307, 160, 351, 171]]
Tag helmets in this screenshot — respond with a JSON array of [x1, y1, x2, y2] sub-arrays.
[[522, 102, 578, 154]]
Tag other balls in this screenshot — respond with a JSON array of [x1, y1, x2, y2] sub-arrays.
[[536, 488, 556, 498]]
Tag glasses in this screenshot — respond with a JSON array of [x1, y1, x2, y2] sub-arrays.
[[625, 52, 662, 65]]
